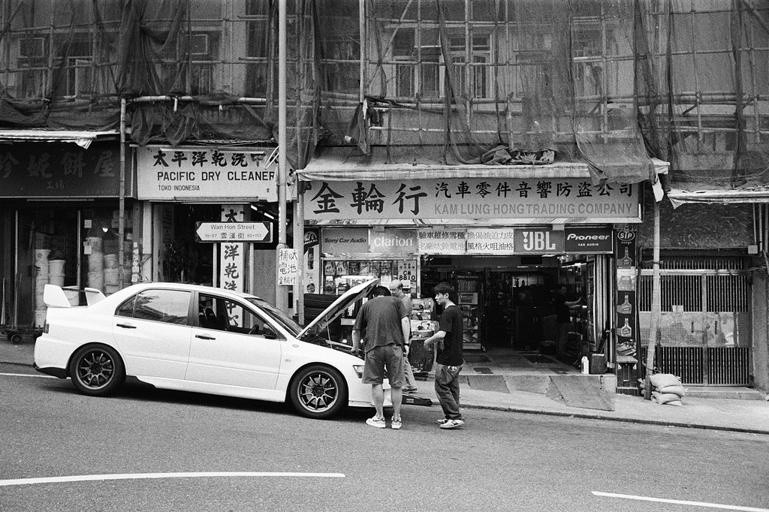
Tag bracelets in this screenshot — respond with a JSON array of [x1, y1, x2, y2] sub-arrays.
[[403, 342, 410, 346]]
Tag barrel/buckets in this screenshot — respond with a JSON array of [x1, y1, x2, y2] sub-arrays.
[[32, 249, 80, 328], [86, 237, 120, 295]]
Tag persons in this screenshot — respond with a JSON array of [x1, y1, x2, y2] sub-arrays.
[[423, 282, 466, 429], [554, 283, 582, 359], [349, 284, 410, 429]]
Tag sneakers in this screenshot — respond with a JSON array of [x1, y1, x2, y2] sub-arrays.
[[435, 417, 464, 429], [402, 386, 418, 395], [366, 416, 386, 428], [389, 416, 402, 429]]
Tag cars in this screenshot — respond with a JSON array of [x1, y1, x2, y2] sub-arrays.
[[34, 278, 404, 419]]
